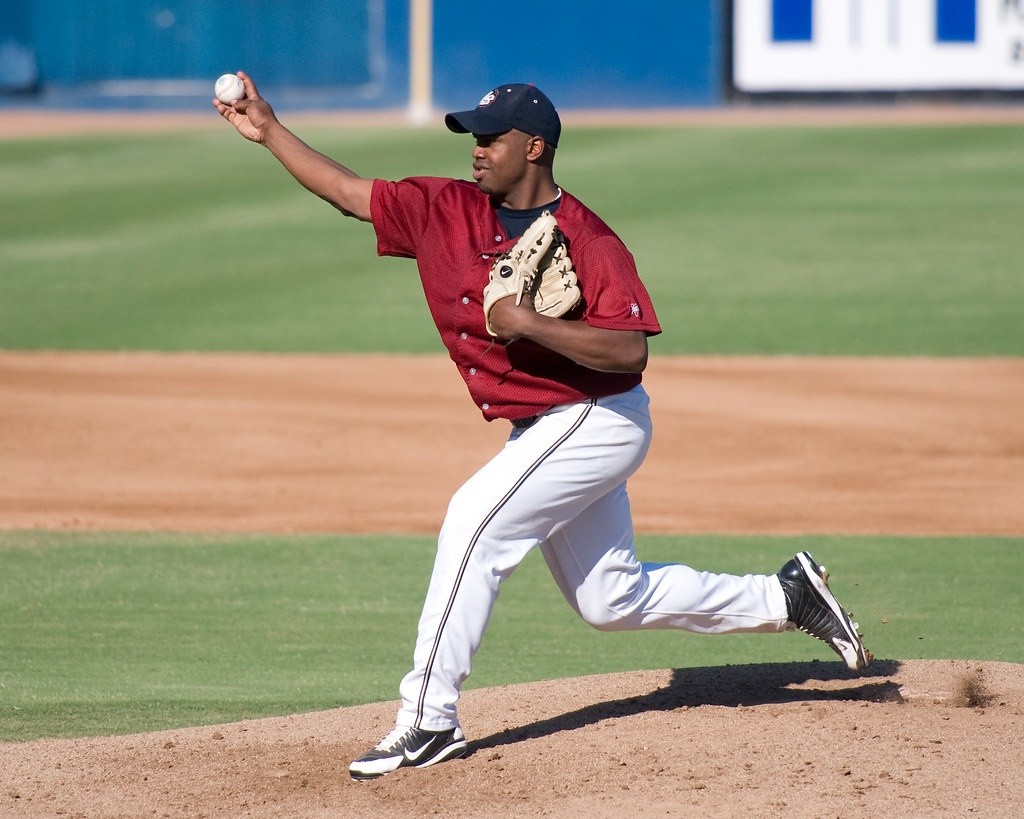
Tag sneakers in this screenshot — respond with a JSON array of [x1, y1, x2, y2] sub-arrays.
[[347, 725, 470, 781], [779, 548, 877, 673]]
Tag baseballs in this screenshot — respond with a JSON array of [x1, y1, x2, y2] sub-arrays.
[[214, 73, 245, 105]]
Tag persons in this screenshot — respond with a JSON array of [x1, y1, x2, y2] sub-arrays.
[[213, 69, 873, 781]]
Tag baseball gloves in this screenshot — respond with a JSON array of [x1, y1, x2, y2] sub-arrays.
[[482, 209, 583, 337]]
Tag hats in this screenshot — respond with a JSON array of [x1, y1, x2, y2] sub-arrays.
[[444, 84, 563, 152]]
